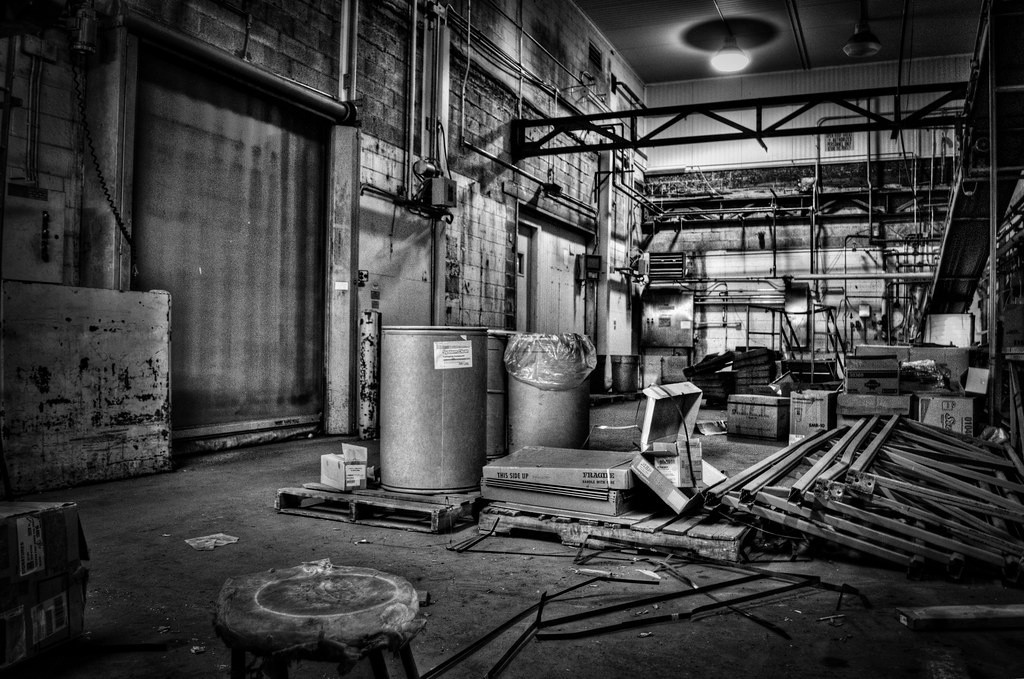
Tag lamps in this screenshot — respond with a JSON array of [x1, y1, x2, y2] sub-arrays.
[[711, 30, 748, 73], [844, 22, 880, 60]]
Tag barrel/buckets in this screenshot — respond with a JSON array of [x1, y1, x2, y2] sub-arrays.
[[591, 354, 607, 394], [506, 335, 592, 454], [380, 326, 486, 494], [610, 355, 641, 393], [487, 329, 514, 459]]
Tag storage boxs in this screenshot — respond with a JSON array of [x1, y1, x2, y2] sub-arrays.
[[0, 502, 91, 671], [321, 443, 368, 491], [727, 346, 974, 443], [483, 382, 727, 514]]
